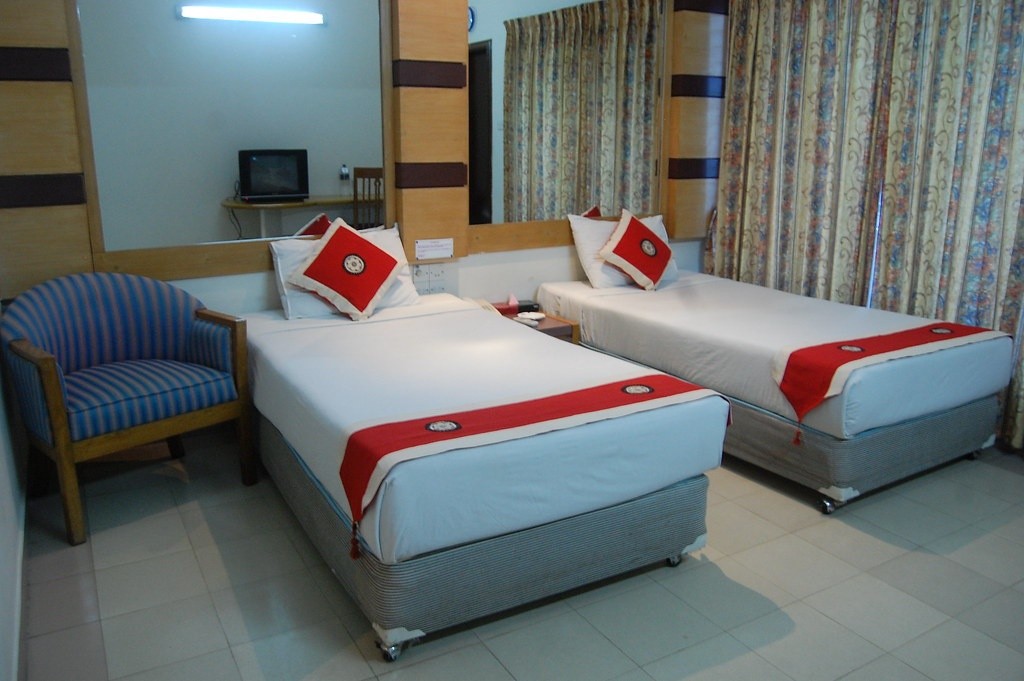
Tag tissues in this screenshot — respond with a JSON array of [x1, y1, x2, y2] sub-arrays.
[[490, 294, 539, 316]]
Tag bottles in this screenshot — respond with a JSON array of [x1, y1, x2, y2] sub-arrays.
[[340, 164, 351, 195]]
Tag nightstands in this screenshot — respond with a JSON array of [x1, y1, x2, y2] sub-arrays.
[[501, 308, 582, 347]]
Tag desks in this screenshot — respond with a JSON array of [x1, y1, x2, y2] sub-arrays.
[[220, 192, 386, 239]]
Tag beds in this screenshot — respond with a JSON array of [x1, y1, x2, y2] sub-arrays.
[[236, 291, 733, 663], [535, 264, 1015, 515]]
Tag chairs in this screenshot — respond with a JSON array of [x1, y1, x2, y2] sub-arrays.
[[0, 271, 249, 546], [349, 168, 386, 230]]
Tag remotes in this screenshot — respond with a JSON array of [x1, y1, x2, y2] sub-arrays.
[[513, 317, 538, 327]]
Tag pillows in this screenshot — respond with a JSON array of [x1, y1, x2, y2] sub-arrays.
[[566, 213, 680, 291], [267, 222, 423, 319], [288, 217, 407, 320], [580, 206, 602, 218], [599, 208, 673, 291], [293, 213, 332, 236]]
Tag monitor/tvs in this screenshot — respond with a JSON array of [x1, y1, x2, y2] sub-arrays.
[[238, 149, 310, 204]]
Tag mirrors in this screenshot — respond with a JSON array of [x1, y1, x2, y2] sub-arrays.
[[65, 0, 393, 278], [466, 0, 673, 227]]
